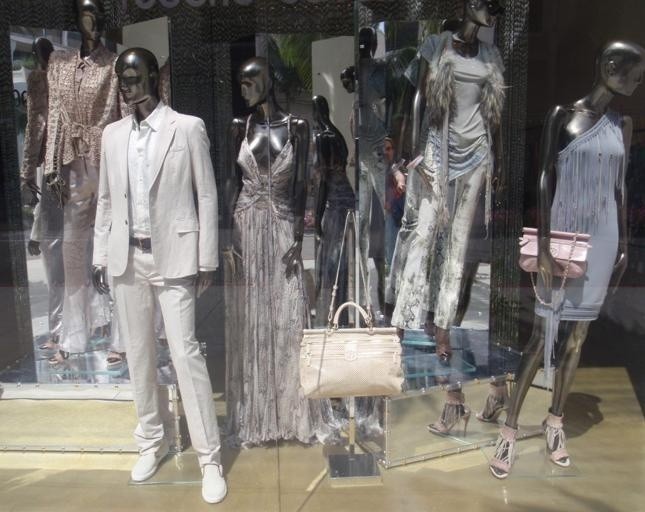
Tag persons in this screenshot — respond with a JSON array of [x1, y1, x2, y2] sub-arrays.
[[490, 39, 645, 479], [93, 47, 227, 504], [20, 1, 506, 448]]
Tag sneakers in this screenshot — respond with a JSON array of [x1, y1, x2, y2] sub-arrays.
[[131, 439, 169, 482], [201, 464, 227, 503]]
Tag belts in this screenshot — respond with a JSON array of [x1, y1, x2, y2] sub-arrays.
[[129, 236, 151, 248]]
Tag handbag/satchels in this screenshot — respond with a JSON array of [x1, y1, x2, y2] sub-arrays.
[[519, 227, 592, 279], [298, 326, 405, 399]]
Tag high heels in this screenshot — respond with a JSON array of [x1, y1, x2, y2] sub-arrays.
[[476, 375, 511, 422], [48, 350, 69, 365], [426, 381, 471, 437], [542, 412, 570, 467], [106, 351, 122, 370], [489, 423, 520, 479], [435, 330, 452, 366]]
